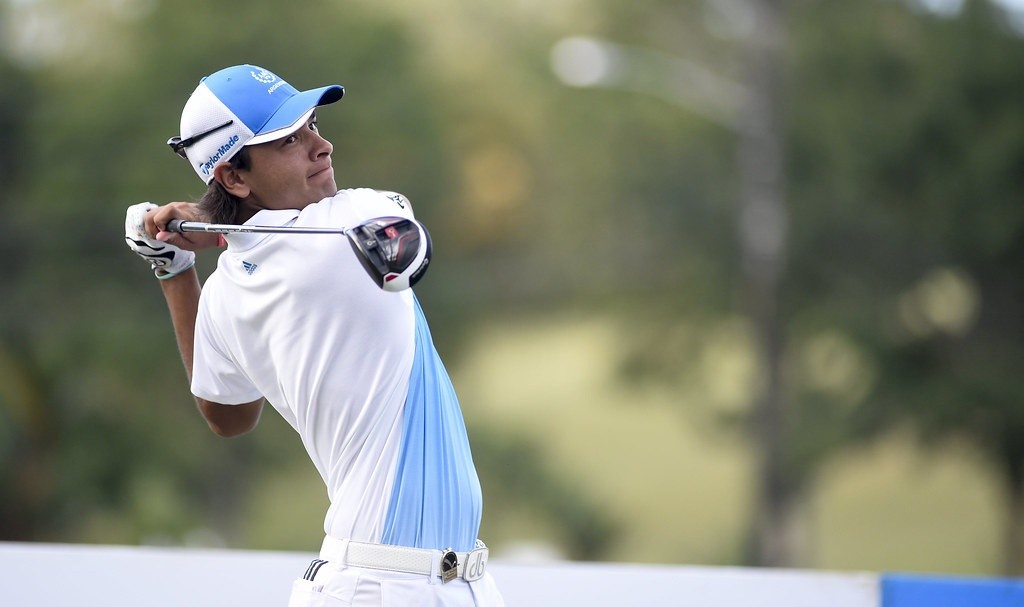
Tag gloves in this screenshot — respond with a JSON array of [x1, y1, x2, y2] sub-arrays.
[[124, 201, 196, 278]]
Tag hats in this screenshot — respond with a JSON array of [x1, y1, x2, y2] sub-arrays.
[[179, 63, 346, 184]]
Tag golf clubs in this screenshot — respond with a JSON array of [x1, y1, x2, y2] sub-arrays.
[[159, 217, 435, 291]]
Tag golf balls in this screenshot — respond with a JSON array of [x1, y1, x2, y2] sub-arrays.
[[552, 35, 607, 89]]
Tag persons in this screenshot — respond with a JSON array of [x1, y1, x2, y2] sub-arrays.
[[124, 61, 508, 607]]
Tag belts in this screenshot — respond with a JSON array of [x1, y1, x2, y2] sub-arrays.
[[319, 535, 490, 584]]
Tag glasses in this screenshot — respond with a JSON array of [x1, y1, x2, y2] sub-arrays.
[[167, 119, 233, 161]]
